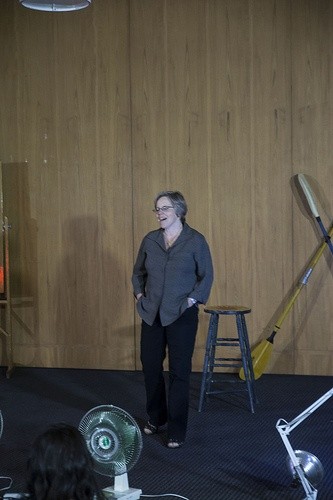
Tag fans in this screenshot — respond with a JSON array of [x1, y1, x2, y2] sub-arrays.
[[77, 405, 144, 500]]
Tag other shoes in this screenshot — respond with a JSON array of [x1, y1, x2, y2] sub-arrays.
[[166, 437, 184, 449], [143, 422, 159, 436]]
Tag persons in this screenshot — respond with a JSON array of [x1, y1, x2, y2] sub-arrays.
[[130, 191, 214, 449], [19, 423, 108, 500]]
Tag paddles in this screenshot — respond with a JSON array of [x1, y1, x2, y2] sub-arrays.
[[239, 218, 333, 383], [292, 171, 333, 256]]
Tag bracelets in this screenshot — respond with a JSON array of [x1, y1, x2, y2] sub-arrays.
[[189, 297, 198, 304]]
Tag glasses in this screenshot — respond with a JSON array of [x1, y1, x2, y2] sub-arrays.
[[152, 205, 174, 212]]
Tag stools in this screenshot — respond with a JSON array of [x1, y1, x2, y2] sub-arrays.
[[198, 305, 258, 413]]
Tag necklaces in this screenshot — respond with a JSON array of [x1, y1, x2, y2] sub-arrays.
[[165, 231, 178, 247]]
